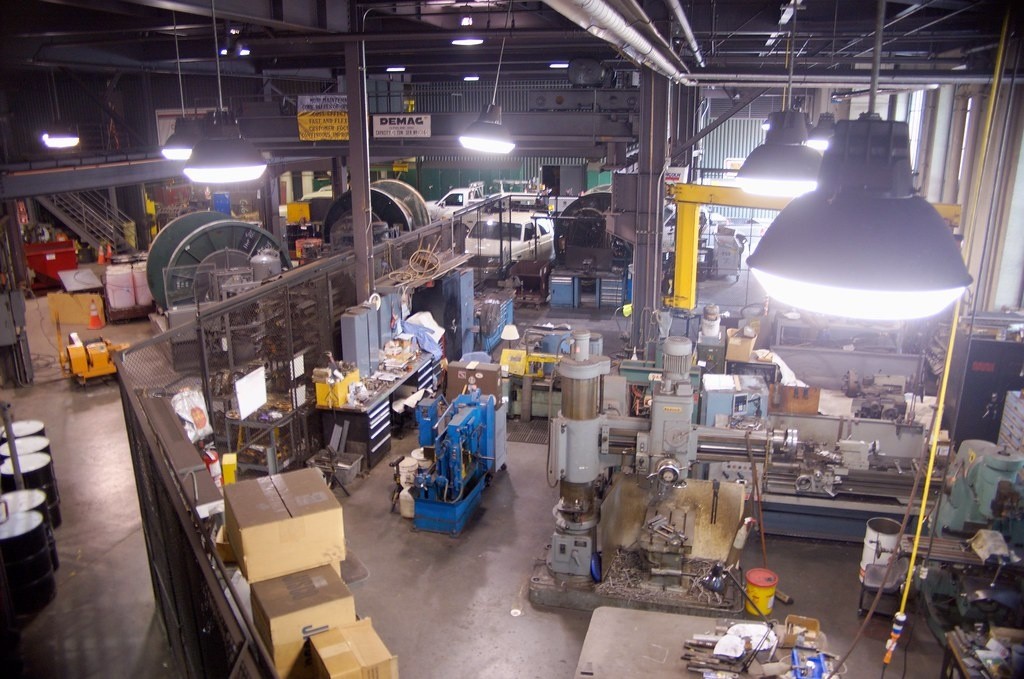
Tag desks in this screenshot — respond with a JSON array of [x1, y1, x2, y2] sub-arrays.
[[940, 631, 1024, 679], [575, 606, 835, 679], [912, 457, 945, 481], [776, 308, 904, 354], [316, 337, 444, 469], [901, 534, 1024, 573]]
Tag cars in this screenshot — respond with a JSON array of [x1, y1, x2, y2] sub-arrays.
[[463, 210, 555, 263]]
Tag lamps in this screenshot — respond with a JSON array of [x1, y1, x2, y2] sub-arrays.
[[501, 324, 519, 350], [183, 0, 267, 183], [734, 0, 972, 321], [459, 0, 515, 154], [550, 47, 569, 69], [464, 72, 479, 81], [43, 67, 79, 148], [686, 562, 773, 674], [386, 66, 406, 72], [220, 46, 251, 57], [161, 11, 204, 160], [452, 0, 483, 46]]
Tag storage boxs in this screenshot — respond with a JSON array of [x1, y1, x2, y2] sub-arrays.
[[446, 360, 502, 410], [726, 328, 757, 363], [216, 449, 400, 679]]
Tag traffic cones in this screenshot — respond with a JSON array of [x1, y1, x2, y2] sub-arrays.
[[105, 243, 112, 265], [86, 297, 106, 330], [97, 243, 106, 265]]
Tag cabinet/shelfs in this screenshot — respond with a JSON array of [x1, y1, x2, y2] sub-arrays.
[[474, 287, 514, 352], [940, 333, 1024, 454], [198, 368, 321, 475], [996, 390, 1024, 483]]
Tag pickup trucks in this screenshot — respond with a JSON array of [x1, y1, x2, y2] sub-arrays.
[[424, 186, 488, 217], [665, 202, 765, 252]]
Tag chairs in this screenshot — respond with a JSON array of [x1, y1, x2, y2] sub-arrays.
[[859, 557, 909, 620]]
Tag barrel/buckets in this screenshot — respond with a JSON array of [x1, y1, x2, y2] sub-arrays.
[[746, 568, 778, 616], [859, 517, 902, 584]]
